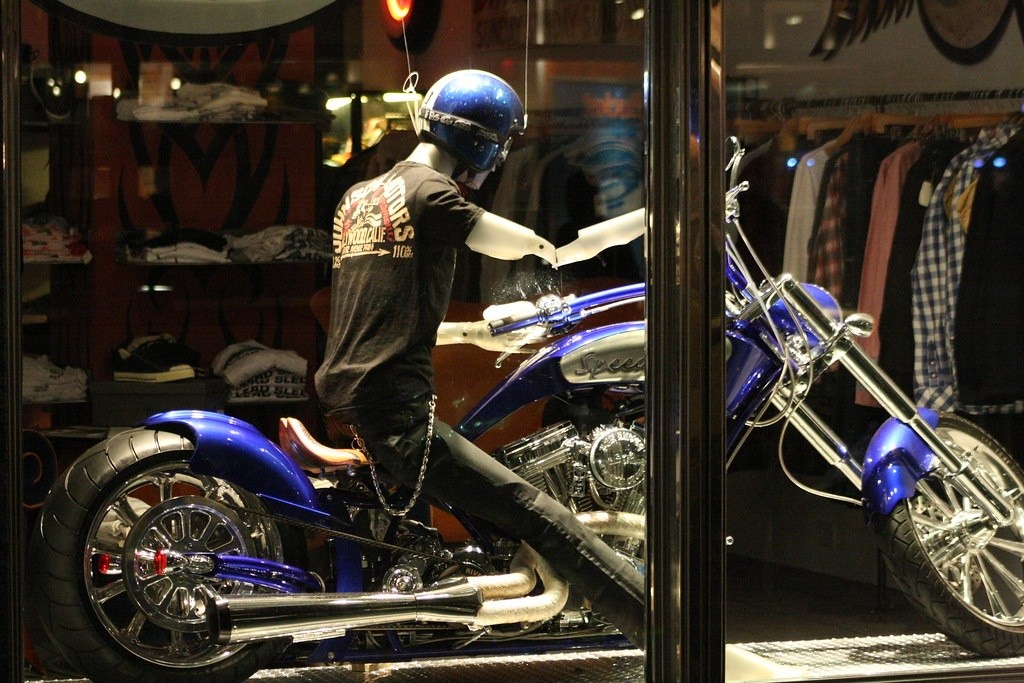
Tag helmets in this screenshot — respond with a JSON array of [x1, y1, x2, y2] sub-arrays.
[[418, 70, 526, 173]]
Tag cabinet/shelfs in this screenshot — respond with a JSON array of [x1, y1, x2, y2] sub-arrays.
[[89, 97, 335, 430], [20, 46, 87, 404]]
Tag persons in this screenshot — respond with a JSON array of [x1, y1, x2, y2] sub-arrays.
[[312, 68, 799, 683]]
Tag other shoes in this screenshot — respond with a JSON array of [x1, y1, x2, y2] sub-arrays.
[[29, 65, 72, 124]]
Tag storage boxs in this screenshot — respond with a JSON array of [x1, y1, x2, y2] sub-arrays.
[[90, 379, 216, 428], [230, 402, 319, 447]]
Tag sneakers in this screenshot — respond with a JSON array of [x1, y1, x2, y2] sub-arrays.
[[111, 344, 195, 383]]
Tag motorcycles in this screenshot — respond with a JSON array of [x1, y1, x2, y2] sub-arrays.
[[24, 137, 1024, 683]]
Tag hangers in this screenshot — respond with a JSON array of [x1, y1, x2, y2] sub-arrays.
[[730, 87, 1024, 155], [511, 106, 644, 154]]
